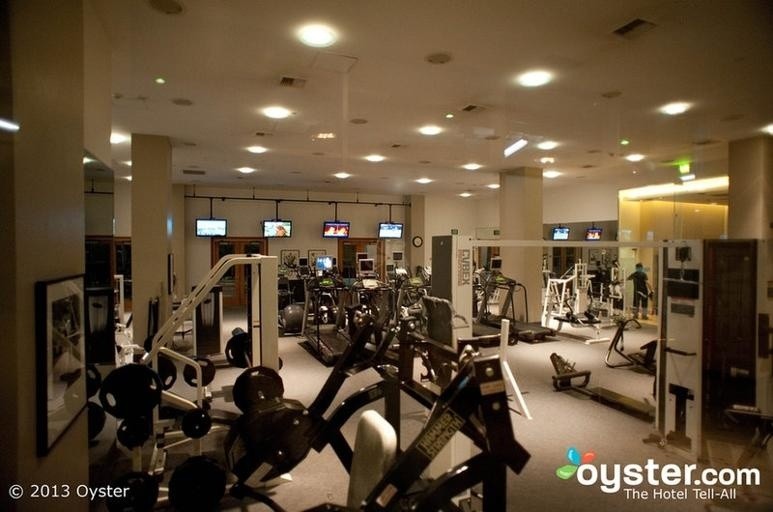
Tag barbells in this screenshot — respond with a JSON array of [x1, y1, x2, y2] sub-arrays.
[[102, 351, 178, 452], [107, 418, 159, 512], [169, 400, 226, 512], [224, 333, 284, 413], [86, 359, 106, 446], [180, 357, 216, 438]]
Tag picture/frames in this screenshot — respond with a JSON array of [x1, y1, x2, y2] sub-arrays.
[[35, 274, 90, 459]]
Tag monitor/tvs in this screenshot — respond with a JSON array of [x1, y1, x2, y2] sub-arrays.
[[490, 257, 502, 270], [299, 251, 404, 274], [378, 223, 403, 239], [263, 220, 292, 238], [552, 228, 570, 240], [322, 222, 350, 238], [195, 219, 227, 237], [585, 229, 602, 240]]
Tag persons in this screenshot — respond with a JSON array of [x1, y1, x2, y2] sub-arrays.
[[275, 225, 288, 236], [626, 263, 652, 319]]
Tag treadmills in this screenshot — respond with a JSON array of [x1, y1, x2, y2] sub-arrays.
[[472, 262, 517, 348], [351, 259, 401, 364], [479, 258, 557, 343], [300, 276, 353, 366]]
